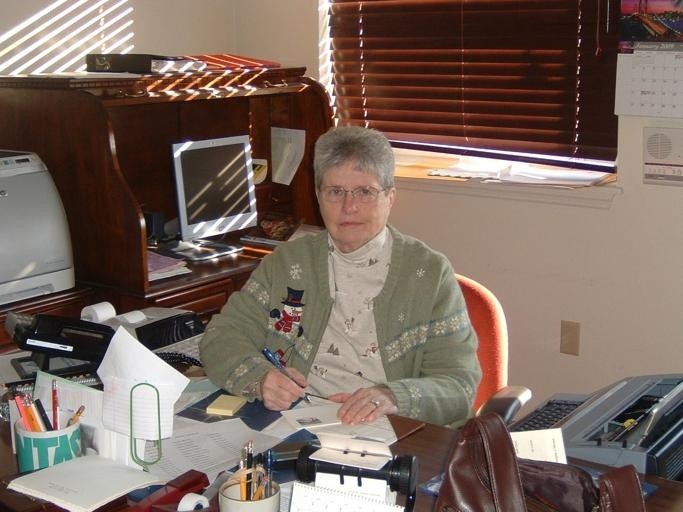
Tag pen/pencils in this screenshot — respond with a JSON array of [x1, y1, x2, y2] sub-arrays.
[[262, 347, 311, 405], [15, 379, 86, 432], [238, 440, 272, 502]]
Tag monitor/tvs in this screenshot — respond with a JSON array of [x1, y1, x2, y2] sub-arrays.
[[169, 134, 259, 262]]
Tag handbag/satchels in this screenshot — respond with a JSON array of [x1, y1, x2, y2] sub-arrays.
[[430, 411, 646, 512]]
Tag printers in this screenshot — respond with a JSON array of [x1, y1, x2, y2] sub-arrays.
[[508, 372, 683, 480]]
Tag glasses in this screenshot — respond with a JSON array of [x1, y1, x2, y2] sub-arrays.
[[319, 185, 389, 205]]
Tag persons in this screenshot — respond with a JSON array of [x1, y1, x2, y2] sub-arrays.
[[198, 126, 482, 430]]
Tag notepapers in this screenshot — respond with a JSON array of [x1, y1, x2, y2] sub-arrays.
[[207, 394, 247, 418]]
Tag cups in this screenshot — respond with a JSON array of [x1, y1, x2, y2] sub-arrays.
[[217, 477, 282, 512], [14, 412, 80, 475]]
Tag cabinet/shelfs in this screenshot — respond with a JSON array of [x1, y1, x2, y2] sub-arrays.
[[0, 67, 335, 330], [0, 287, 96, 356]]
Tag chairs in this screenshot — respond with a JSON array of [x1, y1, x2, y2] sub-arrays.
[[453, 272, 532, 426], [0, 363, 683, 511]]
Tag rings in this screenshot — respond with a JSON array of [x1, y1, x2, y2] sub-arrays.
[[370, 400, 379, 408]]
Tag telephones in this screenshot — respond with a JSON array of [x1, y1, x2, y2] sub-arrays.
[[4, 310, 116, 364]]
